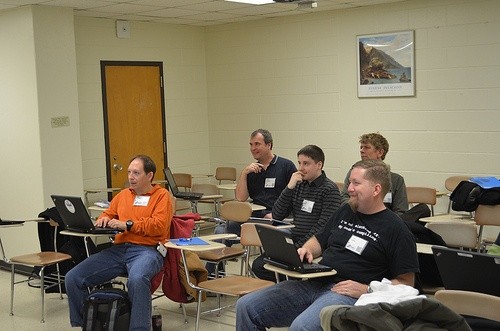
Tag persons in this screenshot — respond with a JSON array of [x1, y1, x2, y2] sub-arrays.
[[251, 145, 341, 282], [65, 155, 174, 331], [205, 129, 298, 296], [236, 160, 419, 331], [341, 133, 409, 217]]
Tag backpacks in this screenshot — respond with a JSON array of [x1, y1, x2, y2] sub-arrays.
[[82, 289, 131, 331]]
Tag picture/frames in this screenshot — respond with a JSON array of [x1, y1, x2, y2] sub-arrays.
[[355, 29, 417, 98]]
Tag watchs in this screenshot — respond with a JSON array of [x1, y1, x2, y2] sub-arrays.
[[367, 284, 373, 293], [126, 220, 133, 231]]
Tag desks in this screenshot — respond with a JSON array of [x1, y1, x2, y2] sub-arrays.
[[0, 183, 337, 331], [419, 211, 475, 223]]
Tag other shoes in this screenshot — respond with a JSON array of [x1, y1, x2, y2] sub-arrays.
[[205, 292, 222, 297]]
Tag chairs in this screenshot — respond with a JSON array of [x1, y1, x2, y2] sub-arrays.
[[0, 165, 276, 331], [405, 175, 500, 331]]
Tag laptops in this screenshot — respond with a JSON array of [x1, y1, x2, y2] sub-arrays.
[[50, 195, 117, 234], [163, 167, 204, 199], [431, 247, 500, 297], [254, 224, 332, 273], [0, 218, 25, 225]]
